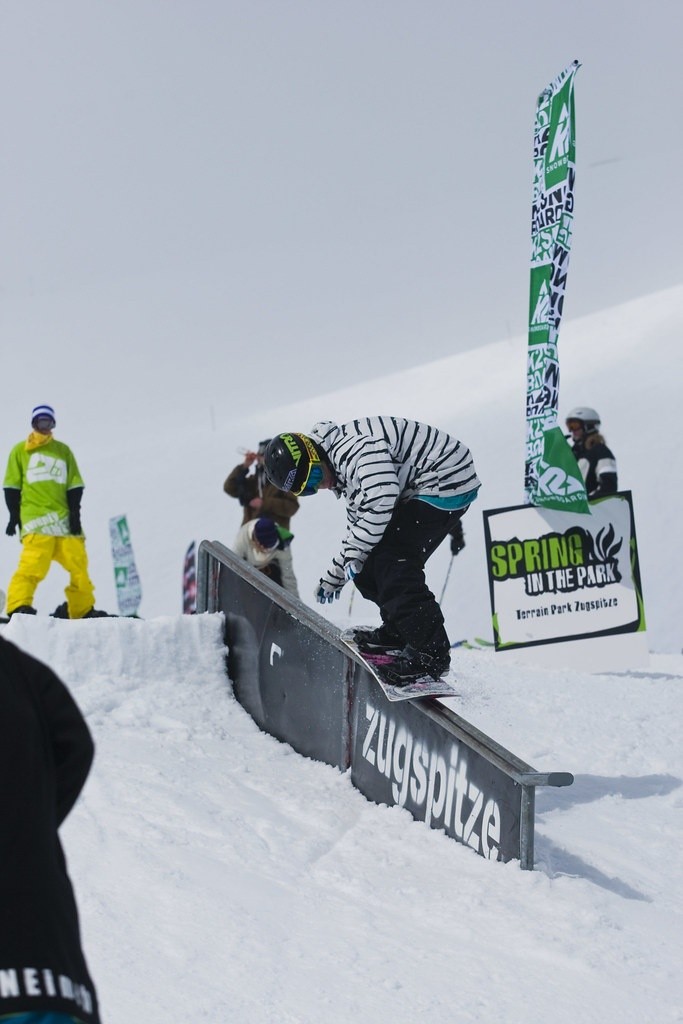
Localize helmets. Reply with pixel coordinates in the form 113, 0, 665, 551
566, 407, 601, 433
264, 432, 325, 497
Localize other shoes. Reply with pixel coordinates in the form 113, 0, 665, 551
83, 610, 107, 618
8, 605, 36, 617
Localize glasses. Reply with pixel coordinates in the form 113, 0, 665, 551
290, 432, 324, 497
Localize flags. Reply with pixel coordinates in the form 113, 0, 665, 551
524, 60, 594, 515
109, 515, 141, 617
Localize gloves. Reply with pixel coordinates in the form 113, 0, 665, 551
6, 519, 21, 536
314, 583, 341, 604
69, 514, 81, 535
343, 559, 362, 581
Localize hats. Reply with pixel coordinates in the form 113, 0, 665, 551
31, 405, 55, 428
255, 518, 278, 543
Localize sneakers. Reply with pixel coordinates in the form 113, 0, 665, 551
352, 622, 409, 650
377, 643, 451, 686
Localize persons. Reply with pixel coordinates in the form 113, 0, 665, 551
224, 438, 299, 598
565, 407, 617, 501
0, 635, 100, 1024
264, 415, 482, 687
3, 405, 107, 620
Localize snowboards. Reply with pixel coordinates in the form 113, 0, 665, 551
338, 624, 462, 703
